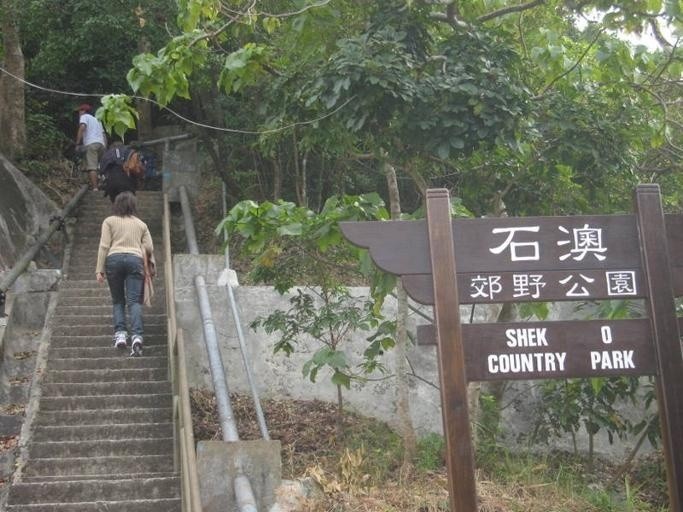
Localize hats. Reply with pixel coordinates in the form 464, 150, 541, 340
75, 104, 92, 112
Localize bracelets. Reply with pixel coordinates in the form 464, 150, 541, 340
76, 143, 82, 146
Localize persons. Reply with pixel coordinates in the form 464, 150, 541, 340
74, 104, 108, 193
94, 190, 154, 357
100, 141, 138, 202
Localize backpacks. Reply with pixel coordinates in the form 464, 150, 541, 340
124, 147, 147, 180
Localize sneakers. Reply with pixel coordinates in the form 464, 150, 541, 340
130, 335, 144, 357
114, 333, 127, 348
